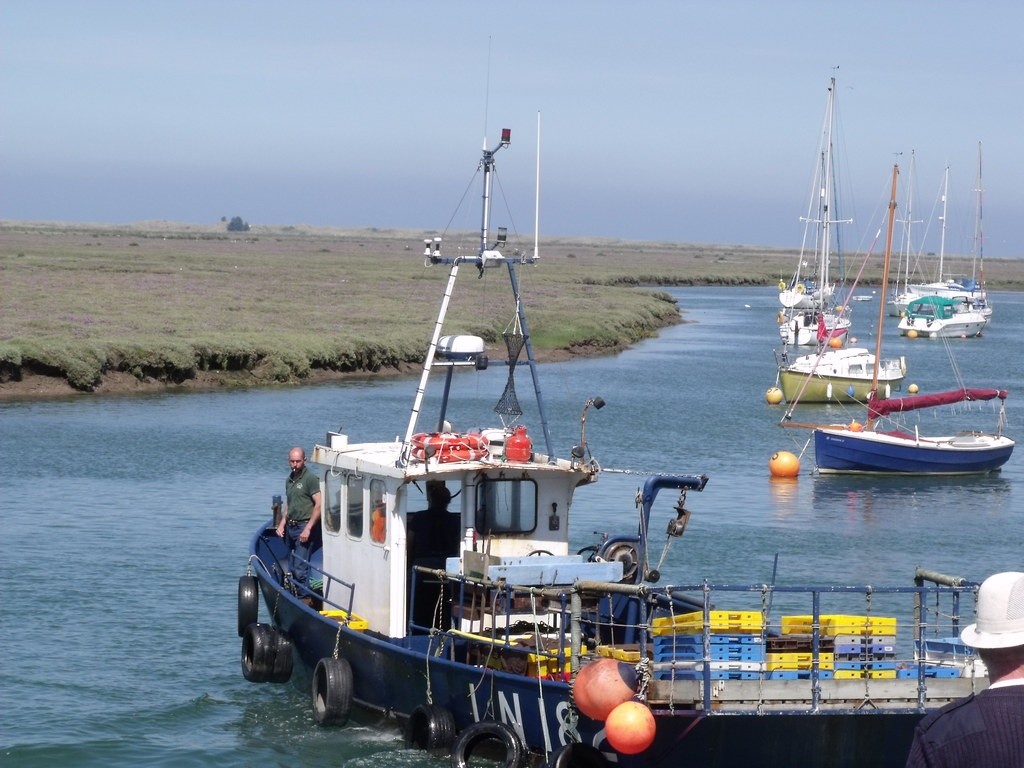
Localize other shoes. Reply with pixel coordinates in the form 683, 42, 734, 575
300, 597, 313, 606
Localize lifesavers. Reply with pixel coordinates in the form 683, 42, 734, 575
410, 448, 488, 462
411, 431, 489, 450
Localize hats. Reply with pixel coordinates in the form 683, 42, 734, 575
959, 572, 1024, 649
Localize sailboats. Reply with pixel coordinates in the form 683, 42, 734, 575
780, 143, 839, 308
891, 162, 990, 337
883, 140, 992, 313
775, 148, 1015, 475
766, 132, 907, 405
779, 77, 854, 348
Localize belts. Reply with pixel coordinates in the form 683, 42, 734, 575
286, 520, 310, 528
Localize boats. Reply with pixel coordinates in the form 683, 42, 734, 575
233, 108, 989, 768
852, 294, 873, 302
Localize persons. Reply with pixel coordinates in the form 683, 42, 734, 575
276, 446, 321, 611
905, 571, 1024, 768
372, 497, 385, 541
408, 484, 460, 598
